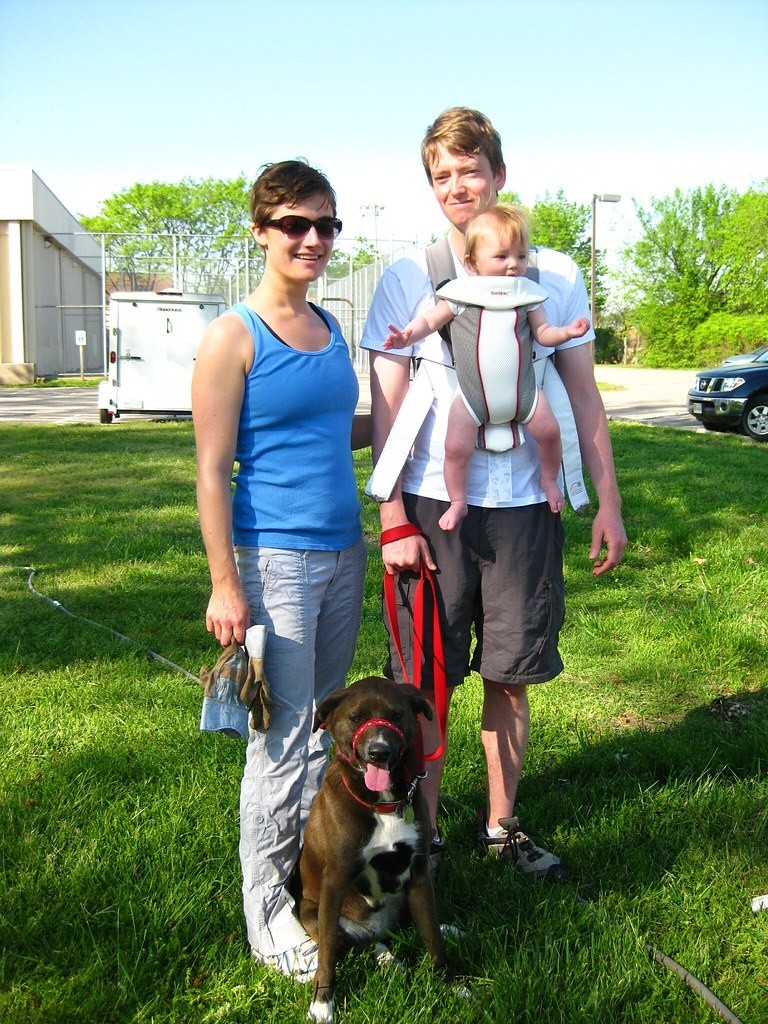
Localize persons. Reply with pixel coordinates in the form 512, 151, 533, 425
383, 205, 592, 532
193, 159, 374, 984
358, 107, 629, 880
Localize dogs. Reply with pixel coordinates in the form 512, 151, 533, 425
295, 673, 474, 1024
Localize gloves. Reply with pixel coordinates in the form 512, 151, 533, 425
200, 637, 252, 740
238, 625, 272, 731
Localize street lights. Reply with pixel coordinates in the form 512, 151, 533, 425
590, 194, 622, 371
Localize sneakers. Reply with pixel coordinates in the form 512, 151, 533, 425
249, 931, 319, 984
475, 817, 566, 880
426, 825, 445, 883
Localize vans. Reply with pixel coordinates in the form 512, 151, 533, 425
99, 287, 228, 425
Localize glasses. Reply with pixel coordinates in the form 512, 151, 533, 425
263, 215, 343, 240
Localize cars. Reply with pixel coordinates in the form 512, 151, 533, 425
721, 346, 768, 372
686, 351, 768, 442
